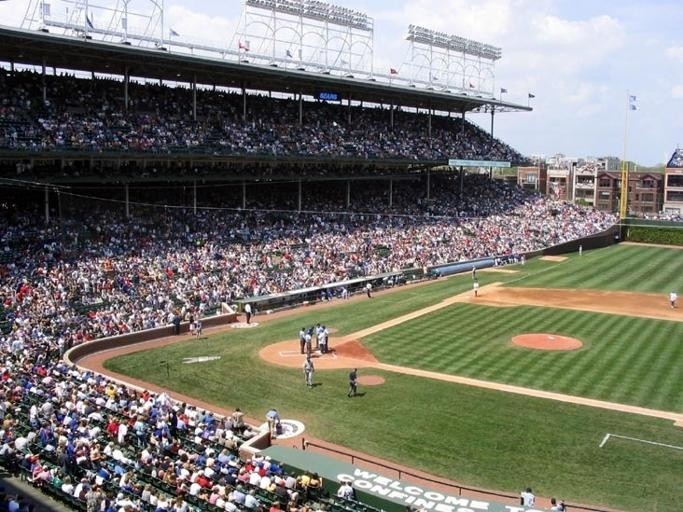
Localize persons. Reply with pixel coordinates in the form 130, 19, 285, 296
643, 211, 683, 222
0, 66, 620, 512
670, 291, 677, 309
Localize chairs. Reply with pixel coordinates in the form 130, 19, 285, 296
0, 67, 621, 512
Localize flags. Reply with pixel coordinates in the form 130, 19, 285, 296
240, 40, 250, 50
343, 58, 348, 64
287, 50, 293, 58
86, 15, 94, 29
170, 28, 180, 37
